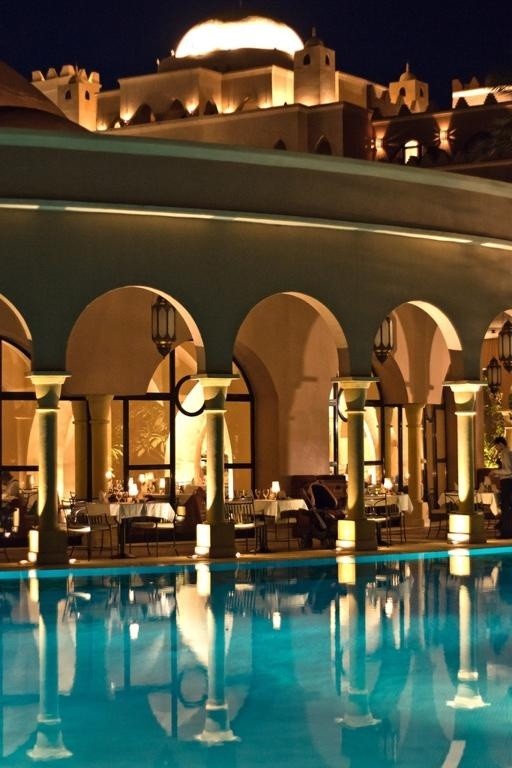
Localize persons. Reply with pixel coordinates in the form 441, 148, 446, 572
0, 471, 19, 528
490, 437, 512, 539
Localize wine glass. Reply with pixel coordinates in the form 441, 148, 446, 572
116, 491, 129, 504
243, 488, 269, 501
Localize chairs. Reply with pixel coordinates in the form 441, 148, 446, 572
224, 499, 266, 556
423, 488, 490, 538
61, 496, 179, 562
362, 492, 402, 548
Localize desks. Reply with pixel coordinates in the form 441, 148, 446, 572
440, 491, 494, 534
363, 492, 408, 543
232, 497, 306, 553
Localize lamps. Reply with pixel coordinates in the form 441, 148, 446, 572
373, 316, 394, 365
151, 294, 177, 359
485, 314, 511, 398
